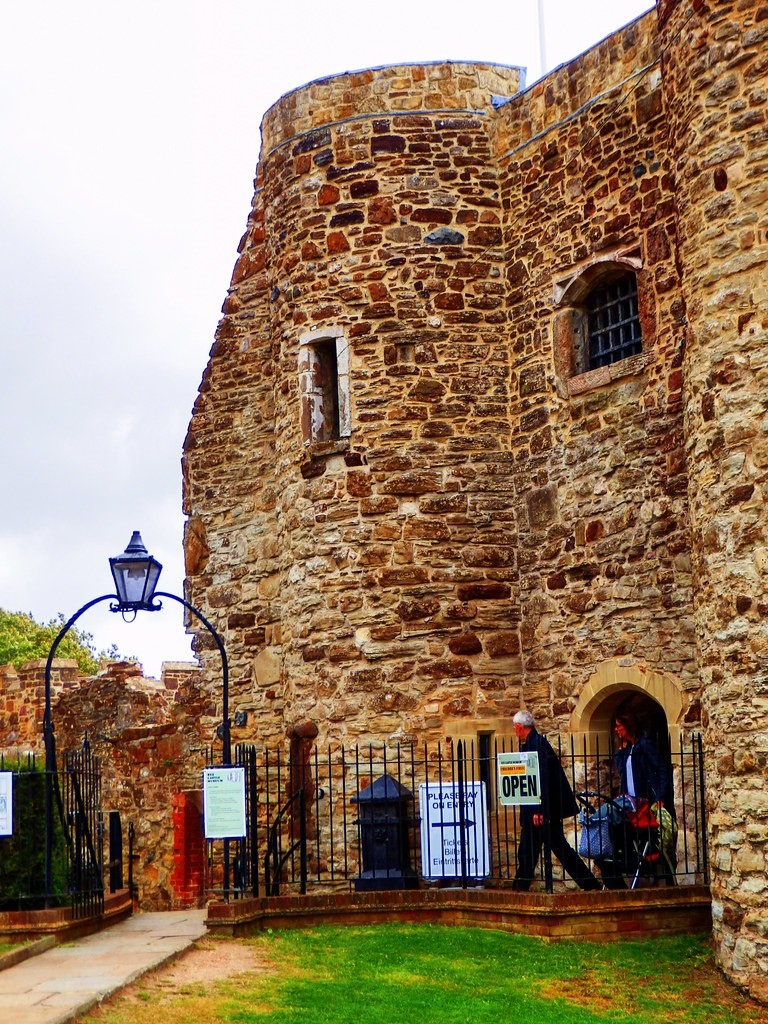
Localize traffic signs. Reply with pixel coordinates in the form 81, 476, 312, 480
419, 781, 490, 877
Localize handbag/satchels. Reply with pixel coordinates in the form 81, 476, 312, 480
651, 802, 678, 852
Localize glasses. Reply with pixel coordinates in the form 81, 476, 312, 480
615, 725, 624, 729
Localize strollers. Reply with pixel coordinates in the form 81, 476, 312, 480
574, 791, 677, 891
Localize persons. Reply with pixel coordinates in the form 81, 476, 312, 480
614, 712, 671, 889
509, 710, 608, 892
